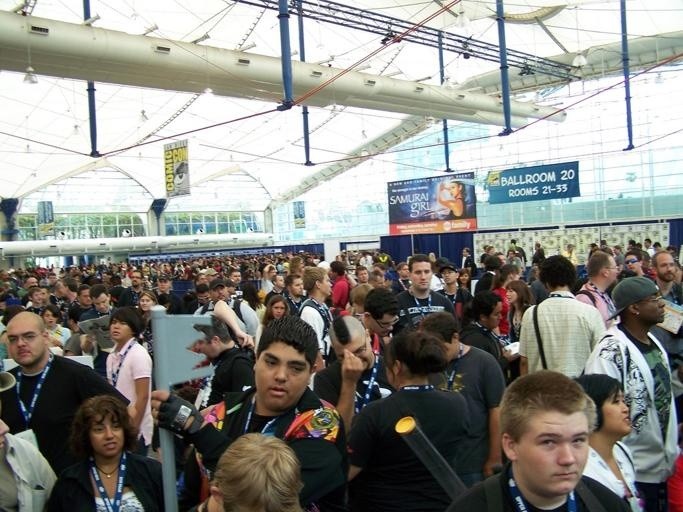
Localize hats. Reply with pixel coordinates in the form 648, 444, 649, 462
207, 278, 225, 290
437, 262, 458, 274
605, 275, 660, 323
203, 268, 219, 277
157, 273, 169, 281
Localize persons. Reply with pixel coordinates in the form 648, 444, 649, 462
438, 181, 466, 220
1, 241, 683, 512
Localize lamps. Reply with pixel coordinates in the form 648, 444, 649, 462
571, 7, 587, 69
457, 0, 469, 30
327, 62, 342, 114
20, 0, 41, 84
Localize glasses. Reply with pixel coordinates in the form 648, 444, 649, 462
332, 332, 367, 363
129, 275, 140, 280
648, 294, 663, 303
623, 257, 640, 265
373, 315, 400, 329
4, 331, 43, 345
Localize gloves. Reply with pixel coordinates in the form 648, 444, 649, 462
156, 392, 206, 438
